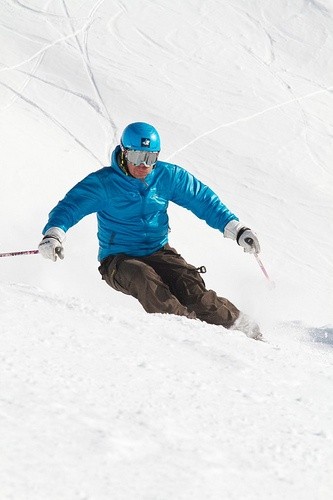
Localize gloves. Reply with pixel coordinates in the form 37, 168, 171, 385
223, 219, 260, 254
38, 227, 66, 262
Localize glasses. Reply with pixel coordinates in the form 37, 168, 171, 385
125, 151, 157, 167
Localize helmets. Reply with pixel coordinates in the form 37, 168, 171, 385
121, 122, 161, 152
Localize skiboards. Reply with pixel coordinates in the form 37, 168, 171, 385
254, 322, 333, 344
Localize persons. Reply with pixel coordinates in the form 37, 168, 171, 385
39, 121, 265, 344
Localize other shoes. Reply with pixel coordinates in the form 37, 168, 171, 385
229, 311, 265, 340
